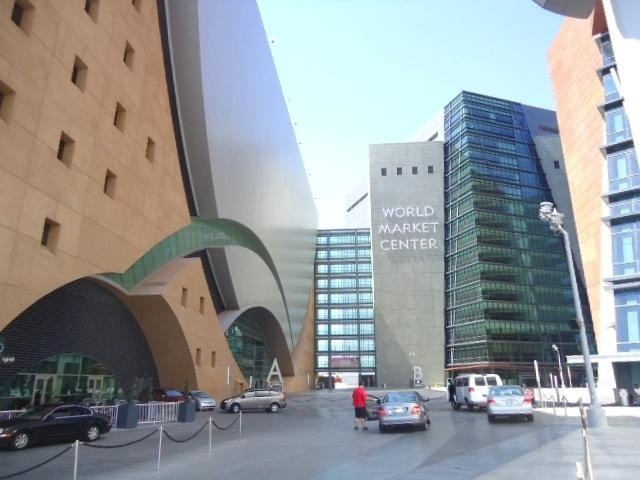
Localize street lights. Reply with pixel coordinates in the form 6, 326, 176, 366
538, 201, 599, 408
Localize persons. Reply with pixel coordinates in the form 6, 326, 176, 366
352, 384, 369, 430
632, 383, 640, 401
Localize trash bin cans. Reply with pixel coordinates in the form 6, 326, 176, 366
614, 387, 633, 407
115, 398, 138, 428
177, 395, 196, 423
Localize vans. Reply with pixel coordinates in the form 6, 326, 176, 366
446, 373, 504, 409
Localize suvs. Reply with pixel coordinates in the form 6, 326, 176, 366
220, 387, 287, 413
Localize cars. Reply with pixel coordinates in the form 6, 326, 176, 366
0, 403, 112, 450
138, 386, 216, 411
482, 385, 534, 422
365, 391, 431, 432
